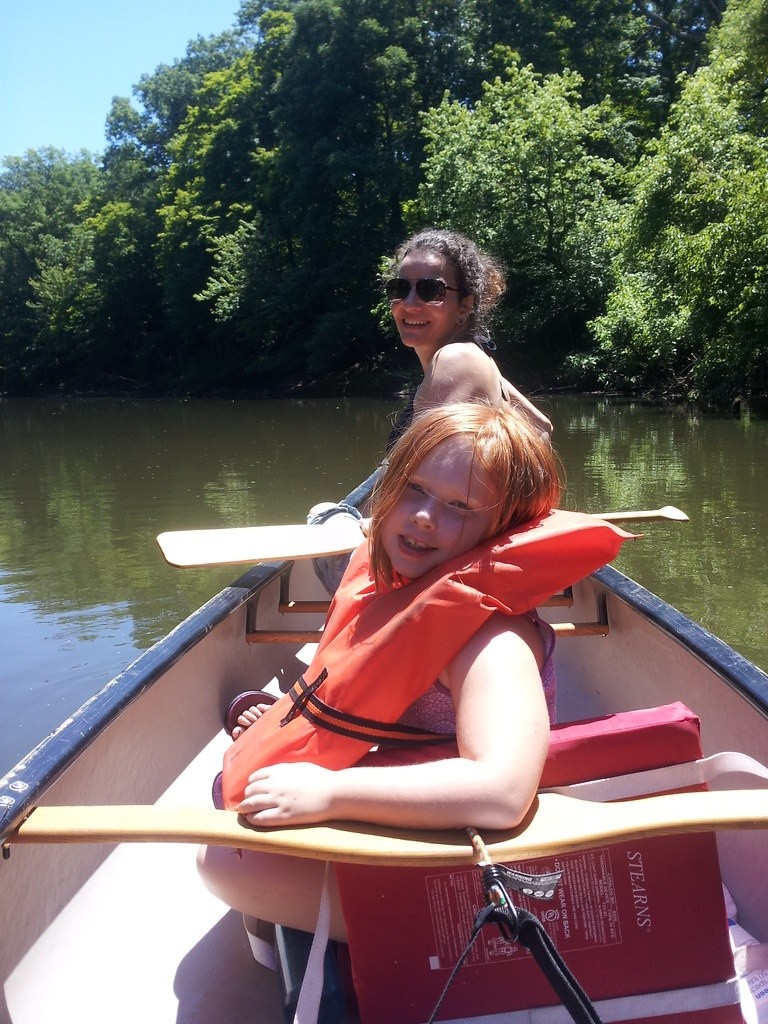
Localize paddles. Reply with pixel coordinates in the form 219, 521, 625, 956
157, 506, 689, 569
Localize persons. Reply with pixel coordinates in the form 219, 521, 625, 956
196, 231, 560, 943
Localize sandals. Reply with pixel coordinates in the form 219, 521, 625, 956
224, 691, 281, 742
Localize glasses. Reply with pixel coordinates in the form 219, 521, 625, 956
384, 279, 462, 304
